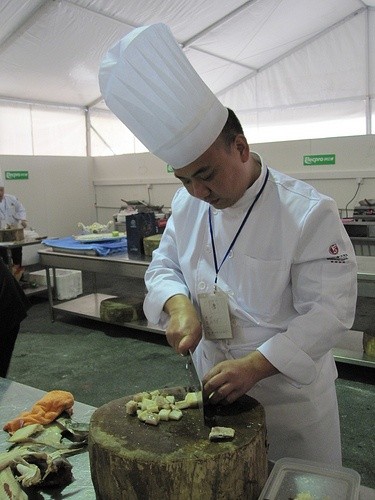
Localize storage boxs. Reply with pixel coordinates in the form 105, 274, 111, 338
143, 234, 164, 262
29, 269, 83, 301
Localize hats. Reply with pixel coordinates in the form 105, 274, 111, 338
98, 23, 229, 170
0, 168, 4, 187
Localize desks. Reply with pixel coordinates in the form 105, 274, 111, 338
37, 248, 375, 369
0, 236, 59, 304
0, 377, 375, 500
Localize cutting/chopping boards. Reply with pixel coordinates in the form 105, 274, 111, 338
99, 296, 145, 323
87, 385, 267, 500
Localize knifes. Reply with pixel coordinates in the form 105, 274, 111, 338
180, 350, 206, 430
